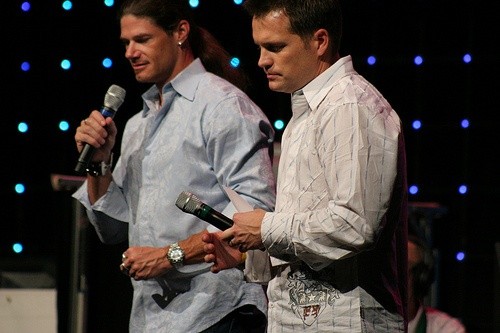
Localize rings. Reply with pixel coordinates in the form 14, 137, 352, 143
121, 263, 129, 271
230, 239, 241, 247
122, 253, 127, 259
84, 118, 88, 125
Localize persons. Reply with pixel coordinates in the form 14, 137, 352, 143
75, 0, 276, 333
202, 0, 404, 333
407, 215, 467, 333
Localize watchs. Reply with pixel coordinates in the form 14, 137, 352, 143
167, 241, 185, 269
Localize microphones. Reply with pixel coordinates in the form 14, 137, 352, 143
73, 84, 126, 177
175, 191, 234, 231
